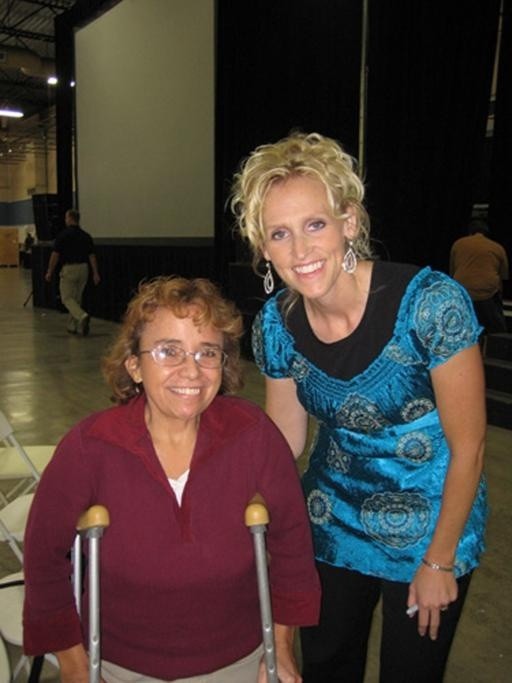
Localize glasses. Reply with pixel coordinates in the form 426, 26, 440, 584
133, 341, 231, 369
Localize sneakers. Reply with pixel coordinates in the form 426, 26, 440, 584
67, 313, 90, 335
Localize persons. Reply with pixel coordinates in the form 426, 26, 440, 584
21, 274, 323, 683
24, 232, 35, 251
45, 209, 102, 337
448, 222, 508, 334
242, 126, 491, 683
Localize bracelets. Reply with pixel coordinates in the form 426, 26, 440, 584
421, 559, 453, 573
